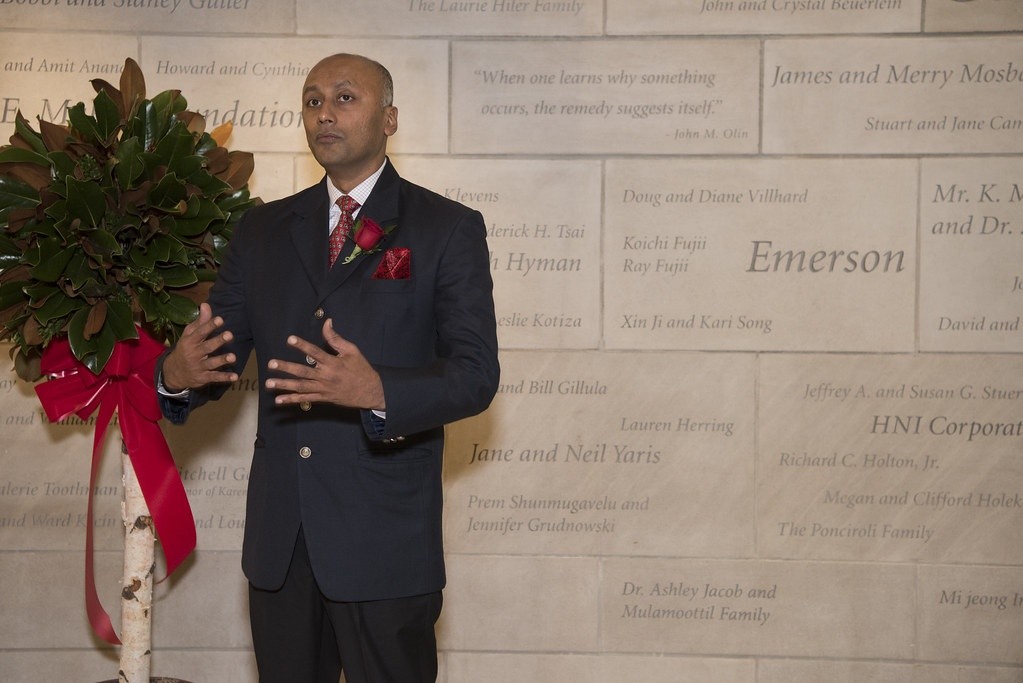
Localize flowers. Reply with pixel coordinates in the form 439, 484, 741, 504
341, 213, 398, 265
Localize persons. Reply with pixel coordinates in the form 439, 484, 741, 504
155, 54, 503, 683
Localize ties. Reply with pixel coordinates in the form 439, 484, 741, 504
329, 196, 361, 272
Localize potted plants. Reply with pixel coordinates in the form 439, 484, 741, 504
0, 54, 270, 683
